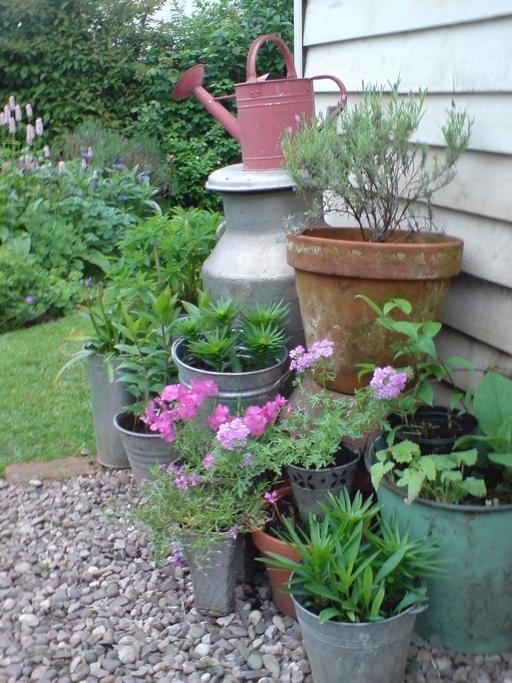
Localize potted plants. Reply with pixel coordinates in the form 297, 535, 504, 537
105, 282, 511, 655
277, 80, 475, 398
254, 485, 453, 683
65, 253, 188, 469
